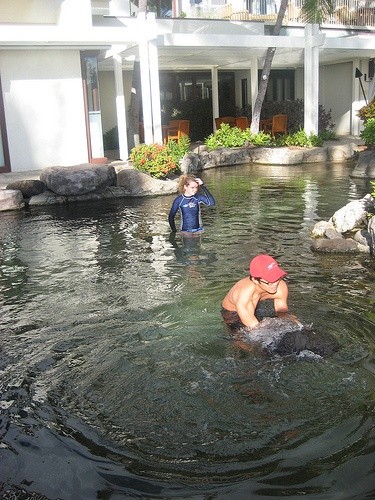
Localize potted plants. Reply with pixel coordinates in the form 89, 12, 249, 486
168, 108, 190, 139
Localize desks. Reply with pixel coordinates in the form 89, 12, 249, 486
139, 125, 177, 145
248, 120, 272, 131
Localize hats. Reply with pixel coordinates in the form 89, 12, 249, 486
250, 255, 287, 283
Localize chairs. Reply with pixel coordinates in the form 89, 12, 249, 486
215, 115, 287, 137
163, 130, 180, 144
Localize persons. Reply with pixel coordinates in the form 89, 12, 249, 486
168, 174, 215, 233
220, 255, 300, 332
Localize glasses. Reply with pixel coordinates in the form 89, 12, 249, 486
259, 280, 272, 286
